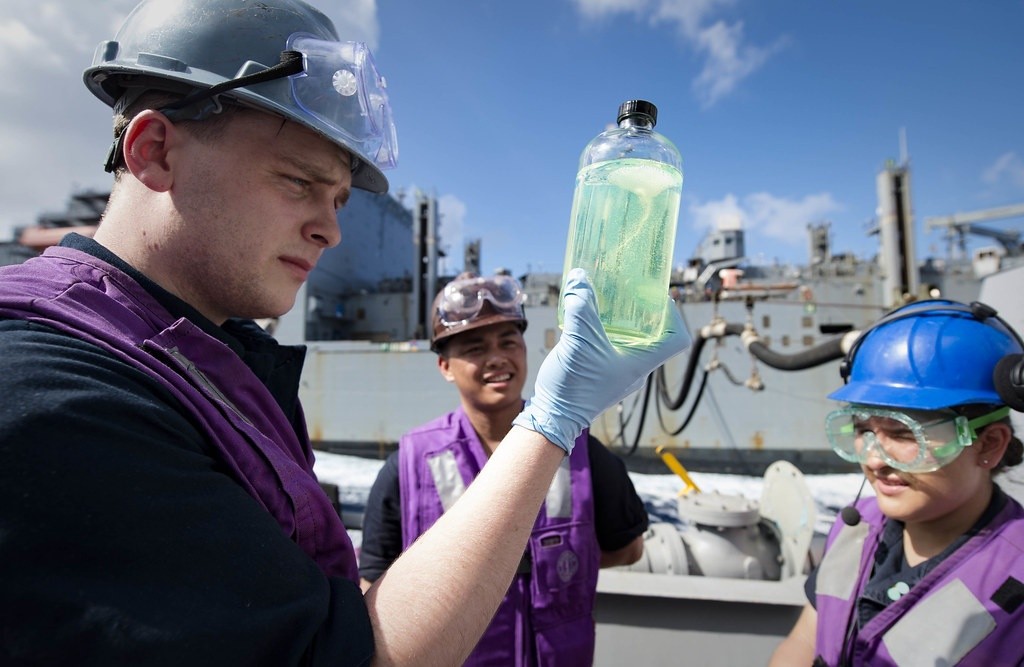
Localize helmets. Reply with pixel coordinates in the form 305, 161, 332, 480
82, 0, 390, 196
429, 272, 530, 353
827, 299, 1024, 413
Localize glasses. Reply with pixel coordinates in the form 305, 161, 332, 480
825, 405, 973, 472
434, 275, 528, 326
287, 32, 399, 169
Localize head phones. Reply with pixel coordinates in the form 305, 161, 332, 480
839, 302, 1024, 413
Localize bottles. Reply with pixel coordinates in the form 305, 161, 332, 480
557, 100, 684, 345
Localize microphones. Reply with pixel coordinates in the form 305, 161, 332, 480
841, 476, 872, 527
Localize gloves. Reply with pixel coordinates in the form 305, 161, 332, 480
510, 269, 692, 455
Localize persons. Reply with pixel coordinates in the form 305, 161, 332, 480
0, 0, 692, 667
358, 272, 650, 667
766, 299, 1024, 667
